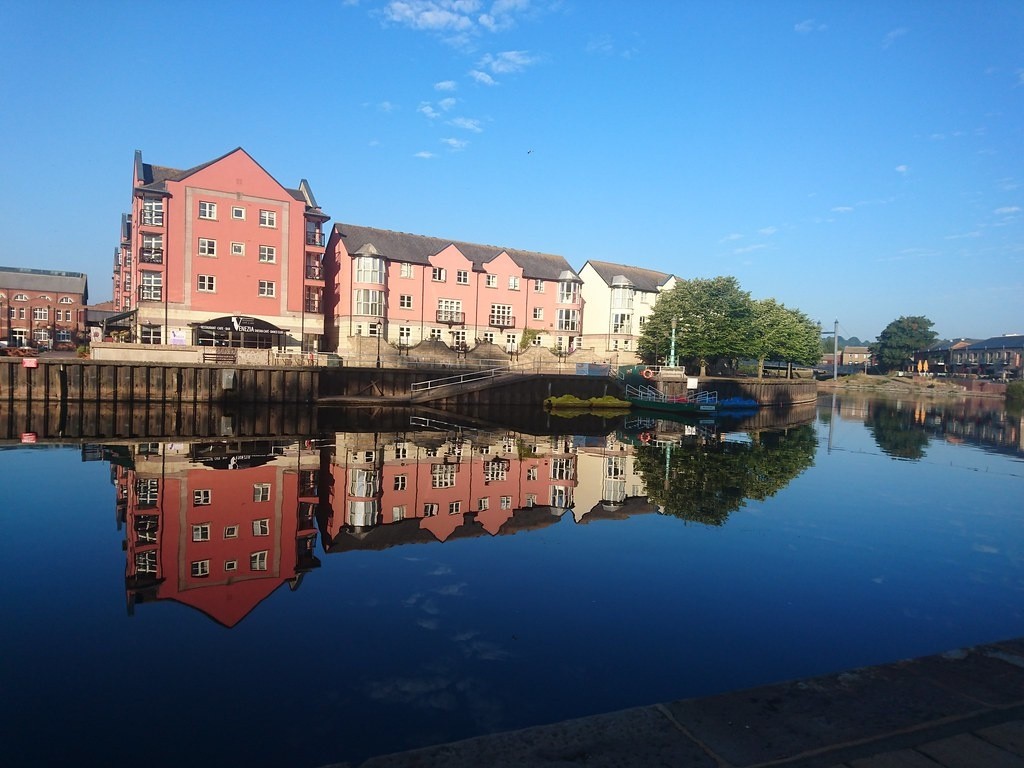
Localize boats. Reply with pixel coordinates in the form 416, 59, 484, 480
587, 395, 632, 407
590, 408, 631, 419
543, 408, 589, 419
543, 394, 592, 408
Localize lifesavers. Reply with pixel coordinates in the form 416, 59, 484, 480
644, 368, 652, 378
640, 432, 651, 441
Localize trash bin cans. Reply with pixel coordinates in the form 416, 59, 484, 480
327, 355, 339, 368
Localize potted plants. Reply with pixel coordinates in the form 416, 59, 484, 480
77, 345, 90, 358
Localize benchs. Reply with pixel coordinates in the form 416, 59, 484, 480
657, 365, 685, 378
203, 353, 237, 364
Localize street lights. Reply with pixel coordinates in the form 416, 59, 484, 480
668, 314, 684, 366
376, 319, 382, 367
